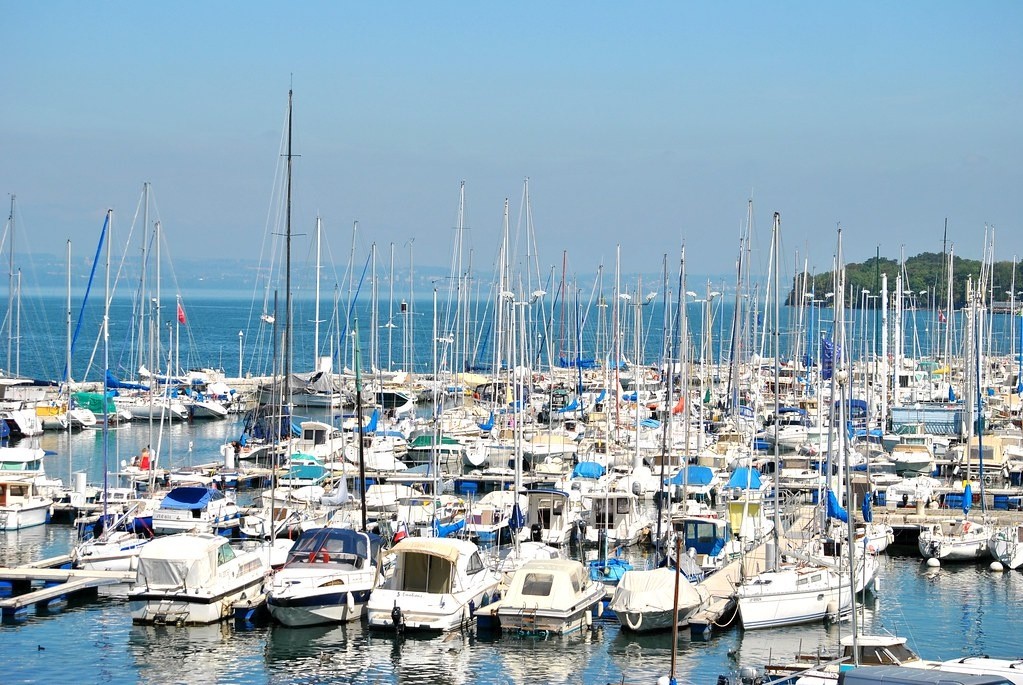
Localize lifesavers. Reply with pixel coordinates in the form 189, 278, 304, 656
309, 548, 329, 563
472, 393, 480, 399
653, 374, 659, 380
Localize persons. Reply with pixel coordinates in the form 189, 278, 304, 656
131, 445, 155, 471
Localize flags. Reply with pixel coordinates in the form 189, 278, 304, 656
703, 389, 710, 402
673, 397, 684, 414
938, 311, 947, 323
179, 305, 185, 325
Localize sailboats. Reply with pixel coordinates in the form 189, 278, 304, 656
0, 71, 1023, 685
